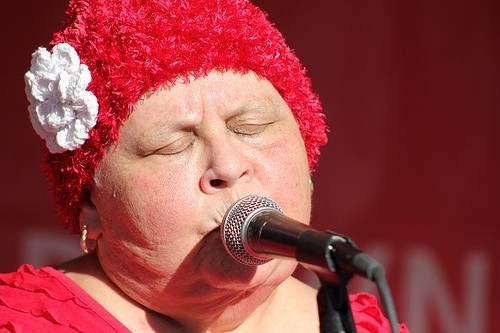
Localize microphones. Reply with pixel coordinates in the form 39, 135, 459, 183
220, 194, 385, 282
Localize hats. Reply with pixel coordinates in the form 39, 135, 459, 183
25, 0, 330, 234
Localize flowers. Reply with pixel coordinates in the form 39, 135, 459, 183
23, 43, 99, 154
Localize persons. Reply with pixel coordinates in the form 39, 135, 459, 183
0, 0, 411, 333
